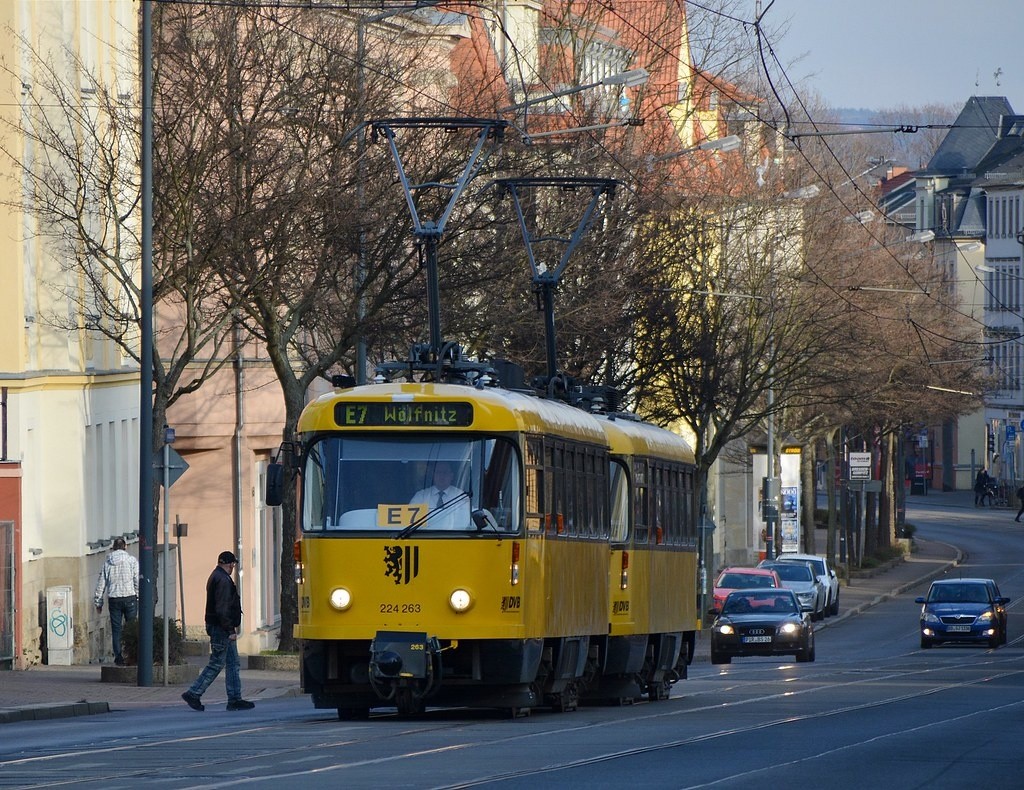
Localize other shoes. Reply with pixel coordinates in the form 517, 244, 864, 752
182, 690, 205, 712
226, 700, 255, 711
1015, 519, 1021, 522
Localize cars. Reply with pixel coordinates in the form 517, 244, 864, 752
707, 588, 816, 664
915, 577, 1012, 649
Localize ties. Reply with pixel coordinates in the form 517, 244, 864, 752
436, 491, 446, 510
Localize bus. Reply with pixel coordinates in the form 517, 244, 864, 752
264, 118, 705, 721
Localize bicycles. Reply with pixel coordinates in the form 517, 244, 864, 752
981, 480, 1009, 508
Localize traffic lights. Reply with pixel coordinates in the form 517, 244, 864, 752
988, 434, 995, 452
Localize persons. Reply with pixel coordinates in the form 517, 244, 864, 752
180, 551, 256, 713
1014, 484, 1024, 522
974, 468, 991, 506
405, 460, 470, 510
774, 598, 787, 611
94, 538, 140, 665
737, 598, 752, 613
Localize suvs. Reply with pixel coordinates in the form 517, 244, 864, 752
710, 564, 784, 622
755, 559, 825, 622
776, 552, 839, 620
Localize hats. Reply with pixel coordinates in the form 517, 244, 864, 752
218, 551, 239, 564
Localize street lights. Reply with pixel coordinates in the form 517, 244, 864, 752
605, 134, 743, 385
765, 209, 874, 561
